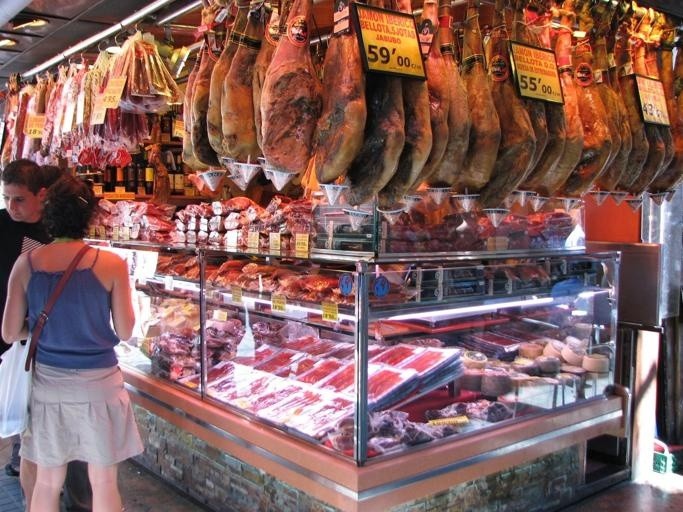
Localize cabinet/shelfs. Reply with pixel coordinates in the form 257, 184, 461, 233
80, 237, 633, 511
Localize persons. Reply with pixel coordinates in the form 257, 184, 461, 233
1, 176, 144, 512
41, 165, 59, 189
0, 158, 92, 512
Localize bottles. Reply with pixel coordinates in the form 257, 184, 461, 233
74, 148, 197, 197
